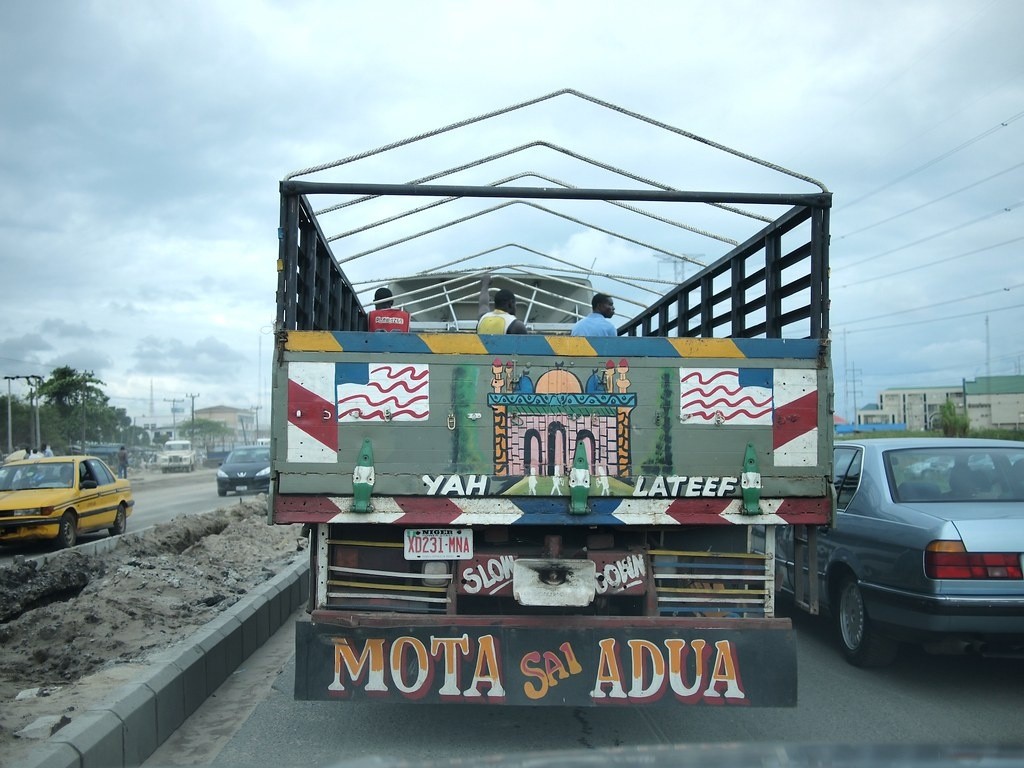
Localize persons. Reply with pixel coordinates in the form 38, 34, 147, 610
362, 288, 410, 333
24, 443, 54, 460
476, 289, 527, 335
58, 466, 72, 487
571, 291, 618, 337
118, 445, 129, 479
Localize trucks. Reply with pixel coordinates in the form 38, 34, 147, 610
262, 175, 838, 708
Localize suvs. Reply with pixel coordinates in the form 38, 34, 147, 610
160, 440, 196, 473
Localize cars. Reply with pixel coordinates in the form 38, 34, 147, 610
750, 439, 1024, 668
0, 456, 135, 548
215, 445, 270, 496
